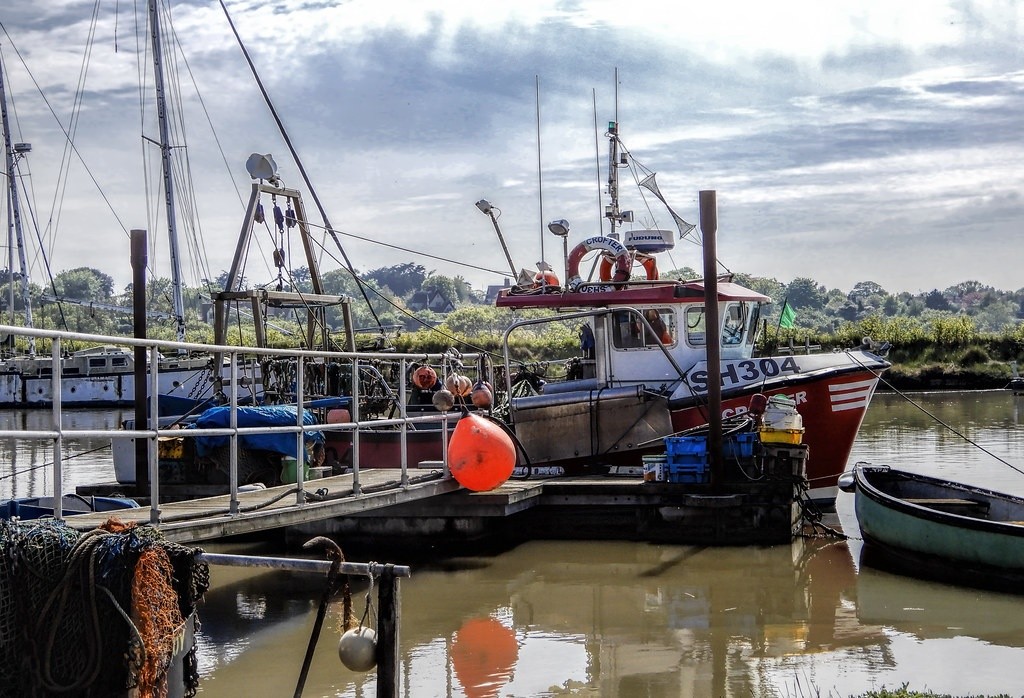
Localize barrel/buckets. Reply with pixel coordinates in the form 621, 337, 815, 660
642, 455, 668, 482
282, 455, 310, 483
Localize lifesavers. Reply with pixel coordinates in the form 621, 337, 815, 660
565, 234, 633, 293
599, 248, 660, 289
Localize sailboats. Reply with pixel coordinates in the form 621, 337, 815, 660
0, 0, 296, 409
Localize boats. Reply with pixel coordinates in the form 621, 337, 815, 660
110, 68, 893, 515
846, 562, 1023, 644
833, 458, 1023, 580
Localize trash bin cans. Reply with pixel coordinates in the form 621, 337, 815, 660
663, 433, 753, 484
